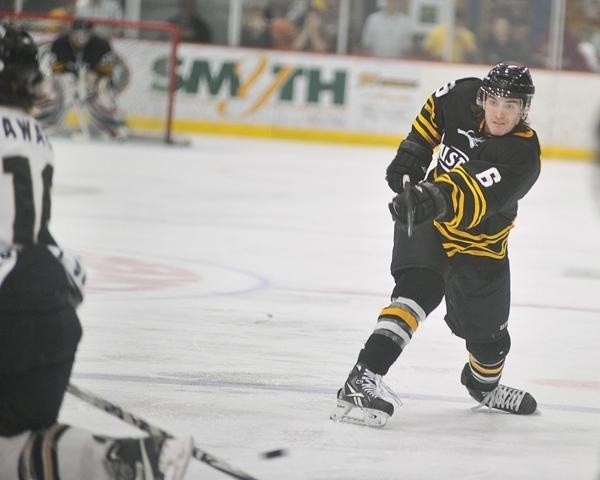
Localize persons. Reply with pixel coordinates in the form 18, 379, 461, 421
336, 61, 543, 416
0, 20, 195, 480
37, 0, 599, 75
29, 15, 132, 141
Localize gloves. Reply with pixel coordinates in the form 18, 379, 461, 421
389, 180, 447, 232
384, 140, 434, 195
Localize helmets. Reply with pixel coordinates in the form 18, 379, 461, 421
479, 60, 535, 111
0, 24, 43, 105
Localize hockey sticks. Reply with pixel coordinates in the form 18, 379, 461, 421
403, 173, 417, 237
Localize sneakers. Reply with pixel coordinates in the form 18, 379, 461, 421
467, 382, 537, 416
336, 363, 395, 419
91, 430, 181, 480
